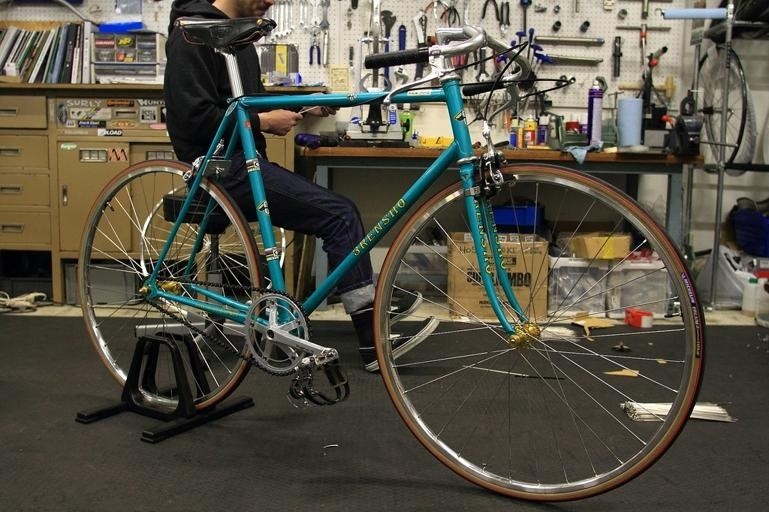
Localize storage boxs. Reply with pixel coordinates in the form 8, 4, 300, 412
446, 204, 668, 324
64, 261, 144, 305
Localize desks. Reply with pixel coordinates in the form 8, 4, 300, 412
300, 143, 705, 317
0, 82, 331, 306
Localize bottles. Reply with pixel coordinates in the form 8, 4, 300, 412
566, 121, 589, 135
509, 116, 550, 147
587, 80, 604, 146
741, 277, 758, 319
399, 104, 414, 142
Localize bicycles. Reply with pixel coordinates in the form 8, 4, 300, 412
75, 16, 707, 502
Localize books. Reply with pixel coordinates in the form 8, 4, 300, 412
0, 21, 100, 85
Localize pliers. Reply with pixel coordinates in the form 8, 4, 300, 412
310, 35, 320, 64
482, 0, 510, 37
424, 0, 462, 28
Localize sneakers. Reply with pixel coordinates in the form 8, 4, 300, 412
358, 290, 440, 372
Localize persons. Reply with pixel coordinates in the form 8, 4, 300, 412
164, 0, 441, 373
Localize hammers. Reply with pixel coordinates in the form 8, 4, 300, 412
618, 75, 673, 98
